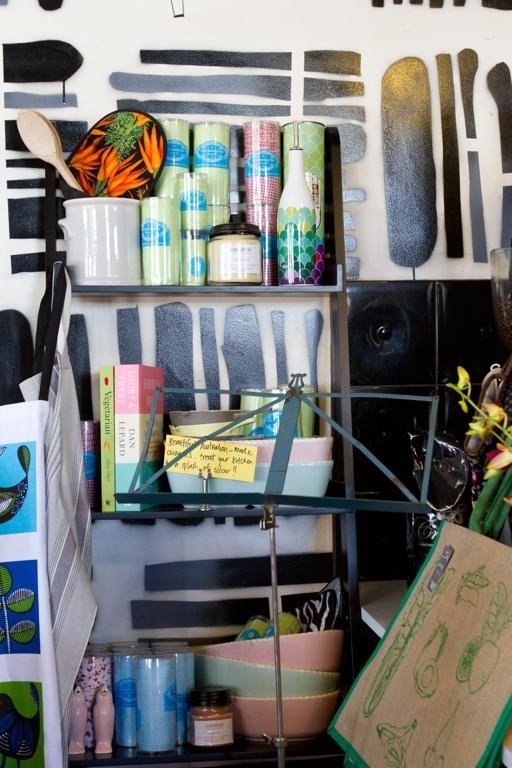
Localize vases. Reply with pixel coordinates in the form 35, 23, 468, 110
276, 149, 316, 286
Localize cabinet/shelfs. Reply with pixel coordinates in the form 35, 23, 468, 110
69, 264, 362, 766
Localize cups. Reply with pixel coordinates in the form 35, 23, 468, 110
54, 198, 141, 289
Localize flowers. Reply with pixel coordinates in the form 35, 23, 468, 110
447, 363, 510, 537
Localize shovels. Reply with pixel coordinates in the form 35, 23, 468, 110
16, 109, 86, 192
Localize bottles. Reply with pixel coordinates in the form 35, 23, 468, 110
237, 610, 298, 639
138, 114, 232, 287
242, 117, 326, 287
241, 387, 317, 438
77, 419, 100, 507
74, 638, 196, 756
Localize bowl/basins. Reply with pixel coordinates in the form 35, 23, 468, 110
166, 407, 272, 438
201, 630, 350, 748
